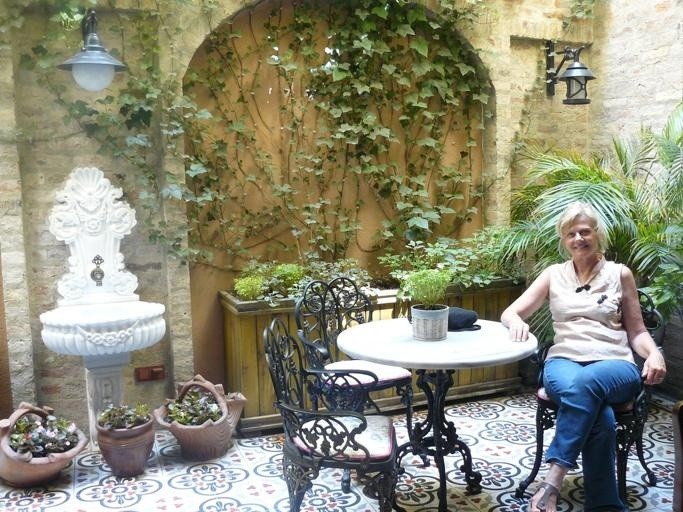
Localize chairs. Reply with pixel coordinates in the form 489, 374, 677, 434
669, 398, 681, 512
513, 286, 668, 508
285, 273, 431, 494
253, 313, 402, 511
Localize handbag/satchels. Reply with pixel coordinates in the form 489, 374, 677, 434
447, 305, 482, 332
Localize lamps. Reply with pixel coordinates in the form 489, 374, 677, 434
541, 37, 598, 107
49, 5, 132, 95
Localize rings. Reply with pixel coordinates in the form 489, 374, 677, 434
657, 377, 664, 380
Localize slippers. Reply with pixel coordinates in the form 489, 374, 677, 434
526, 481, 561, 512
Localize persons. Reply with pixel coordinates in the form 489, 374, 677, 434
500, 200, 667, 512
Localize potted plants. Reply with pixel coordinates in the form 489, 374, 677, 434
396, 267, 451, 344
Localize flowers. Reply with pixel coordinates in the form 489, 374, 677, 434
6, 412, 79, 457
93, 396, 151, 434
162, 387, 222, 426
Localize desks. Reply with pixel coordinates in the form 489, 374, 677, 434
330, 305, 536, 510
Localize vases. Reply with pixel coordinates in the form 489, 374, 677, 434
151, 372, 248, 461
93, 410, 157, 477
0, 399, 90, 489
